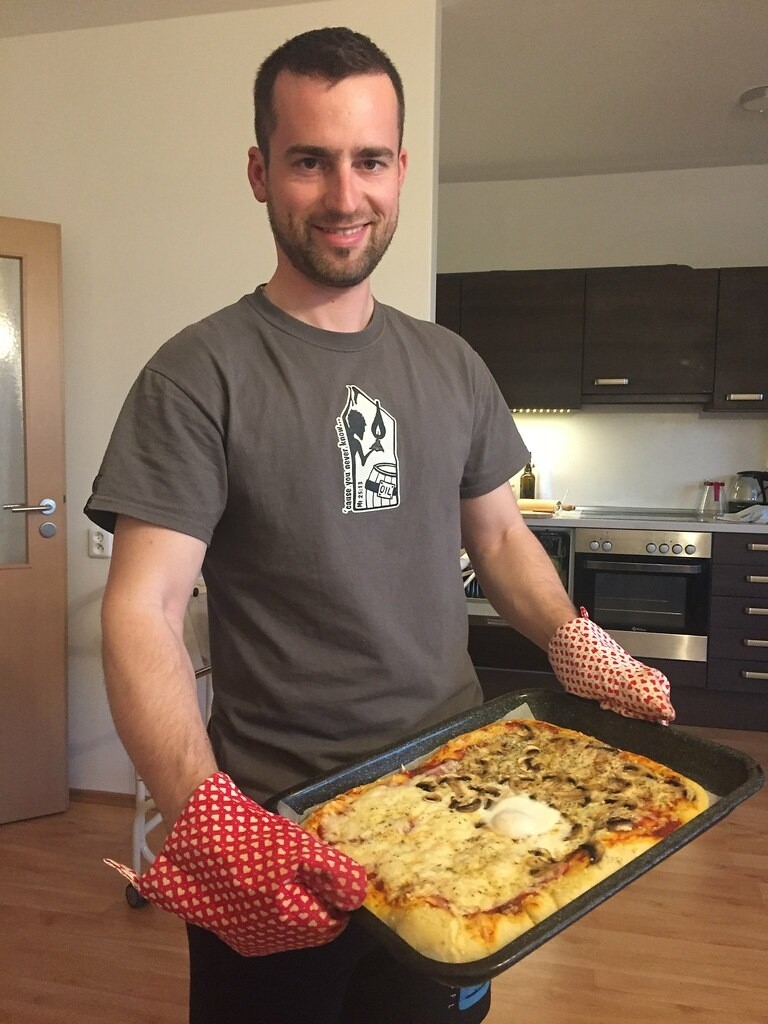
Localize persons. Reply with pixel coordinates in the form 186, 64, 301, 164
83, 26, 678, 1024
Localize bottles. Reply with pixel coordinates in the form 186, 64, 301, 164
520, 452, 535, 499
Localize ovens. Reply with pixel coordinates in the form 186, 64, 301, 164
574, 527, 712, 661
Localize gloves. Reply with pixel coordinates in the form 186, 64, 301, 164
547, 606, 676, 727
105, 772, 368, 957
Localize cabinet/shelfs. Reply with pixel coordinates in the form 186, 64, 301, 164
713, 267, 768, 410
581, 268, 719, 394
461, 272, 586, 410
707, 531, 768, 694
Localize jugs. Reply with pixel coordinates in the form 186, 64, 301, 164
697, 479, 728, 523
729, 471, 768, 513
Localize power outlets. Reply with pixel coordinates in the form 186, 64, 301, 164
88, 527, 113, 559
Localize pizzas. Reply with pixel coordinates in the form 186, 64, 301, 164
298, 719, 708, 962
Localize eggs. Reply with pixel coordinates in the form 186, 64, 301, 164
475, 795, 560, 838
